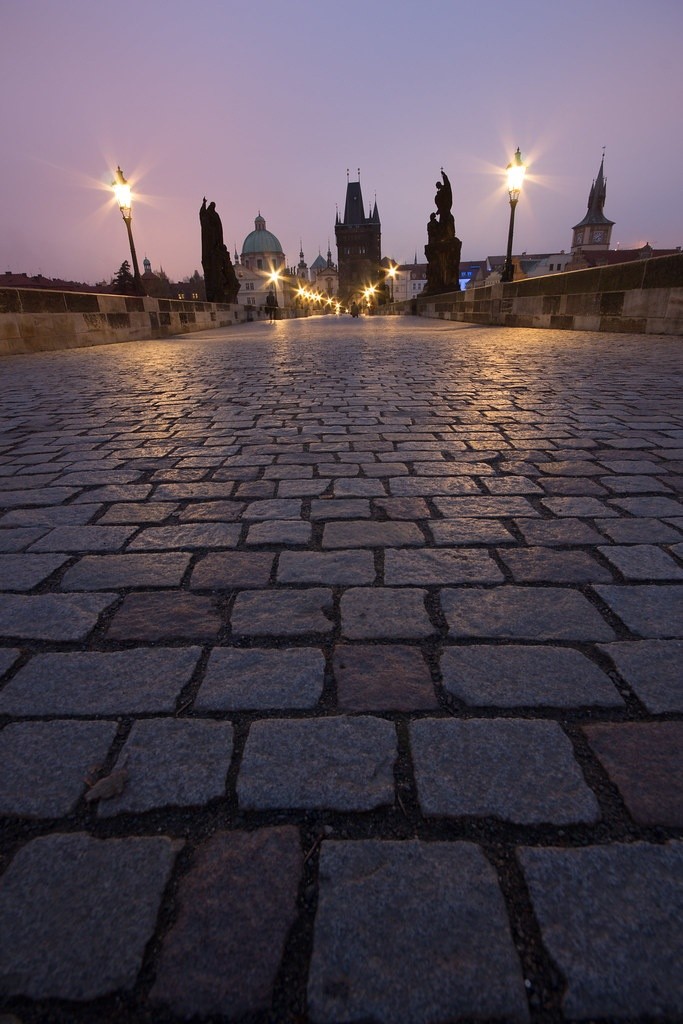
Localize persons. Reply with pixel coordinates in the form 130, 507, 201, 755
351, 302, 360, 318
427, 170, 455, 245
410, 295, 417, 315
266, 290, 279, 320
199, 196, 242, 303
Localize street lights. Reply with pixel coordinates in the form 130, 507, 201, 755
500, 147, 527, 283
272, 271, 279, 307
389, 267, 396, 302
112, 165, 147, 296
